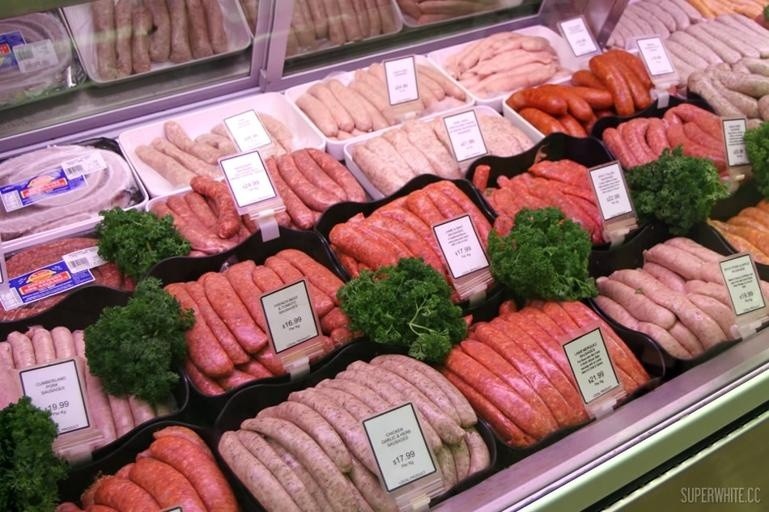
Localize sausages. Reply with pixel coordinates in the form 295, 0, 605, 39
2, 0, 769, 511
